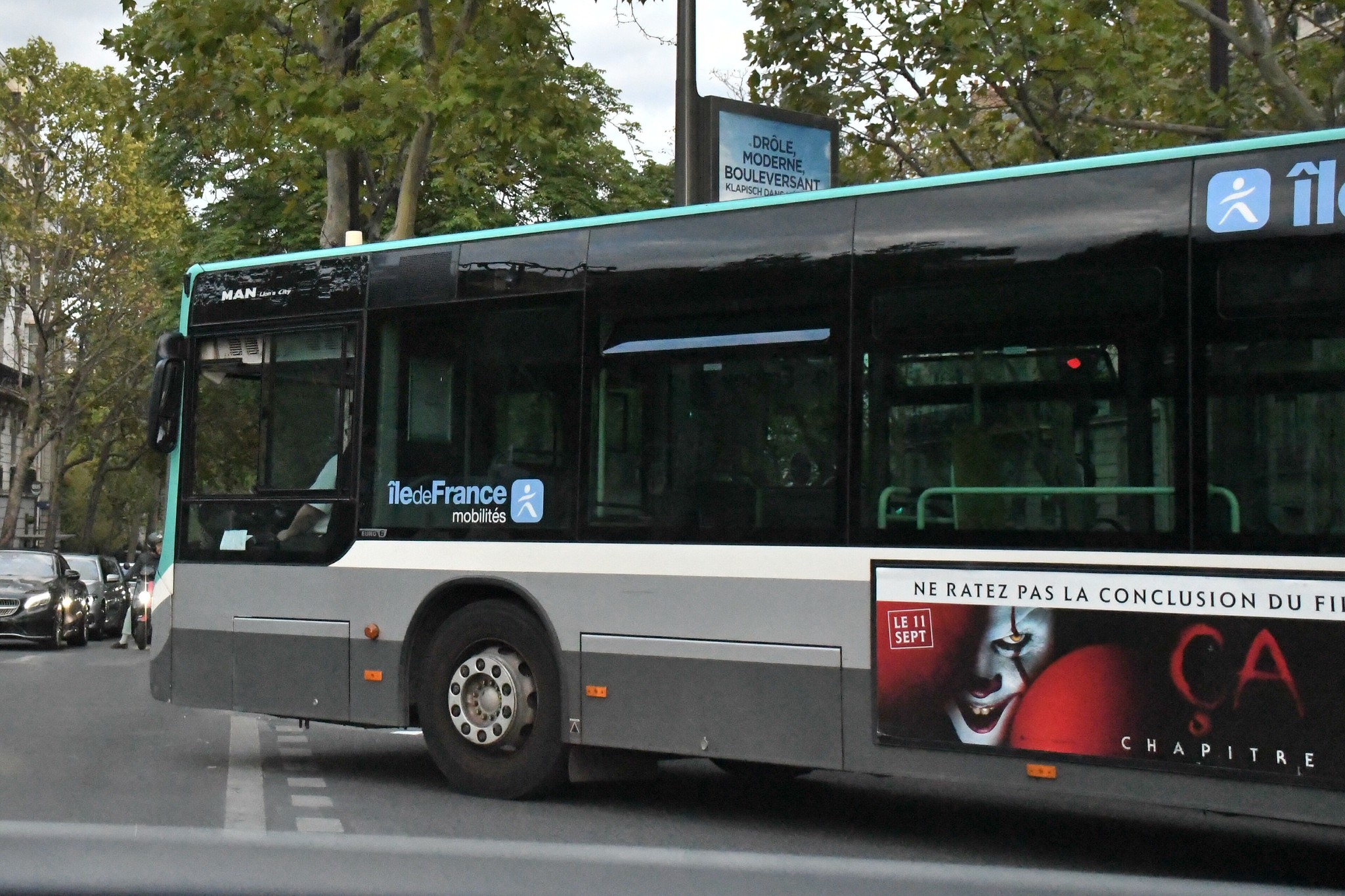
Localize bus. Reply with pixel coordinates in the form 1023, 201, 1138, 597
145, 124, 1344, 859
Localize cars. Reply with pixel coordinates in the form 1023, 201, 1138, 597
0, 547, 136, 649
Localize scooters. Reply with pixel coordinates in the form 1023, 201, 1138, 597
131, 564, 153, 648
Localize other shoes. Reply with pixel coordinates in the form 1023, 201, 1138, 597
110, 641, 129, 649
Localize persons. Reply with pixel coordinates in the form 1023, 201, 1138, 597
112, 533, 162, 650
277, 455, 337, 550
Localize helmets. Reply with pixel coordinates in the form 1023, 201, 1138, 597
146, 532, 164, 551
